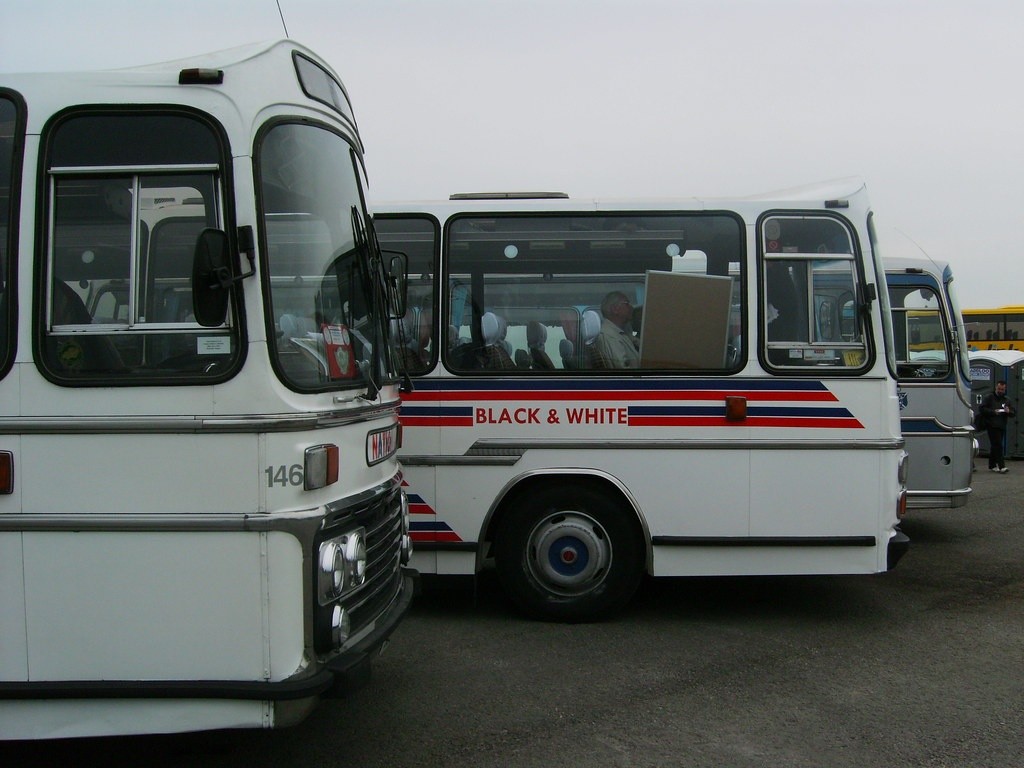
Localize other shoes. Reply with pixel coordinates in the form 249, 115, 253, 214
999, 467, 1010, 474
992, 467, 1001, 473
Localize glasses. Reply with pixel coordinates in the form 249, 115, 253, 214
618, 301, 632, 305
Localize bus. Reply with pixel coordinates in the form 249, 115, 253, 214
83, 256, 980, 513
130, 192, 911, 619
0, 37, 415, 742
905, 304, 1024, 367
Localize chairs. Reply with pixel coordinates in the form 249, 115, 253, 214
986, 329, 992, 340
1008, 329, 1012, 339
281, 315, 310, 371
580, 311, 613, 370
514, 348, 531, 371
481, 312, 517, 372
974, 332, 980, 340
966, 330, 973, 340
526, 320, 555, 371
559, 339, 577, 369
1014, 331, 1018, 339
993, 331, 999, 340
297, 317, 325, 371
504, 340, 513, 357
388, 315, 422, 373
460, 337, 477, 371
447, 325, 459, 370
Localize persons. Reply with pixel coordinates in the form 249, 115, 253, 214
594, 290, 641, 368
978, 380, 1015, 472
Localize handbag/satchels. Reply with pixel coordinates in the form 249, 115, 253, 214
974, 413, 987, 433
